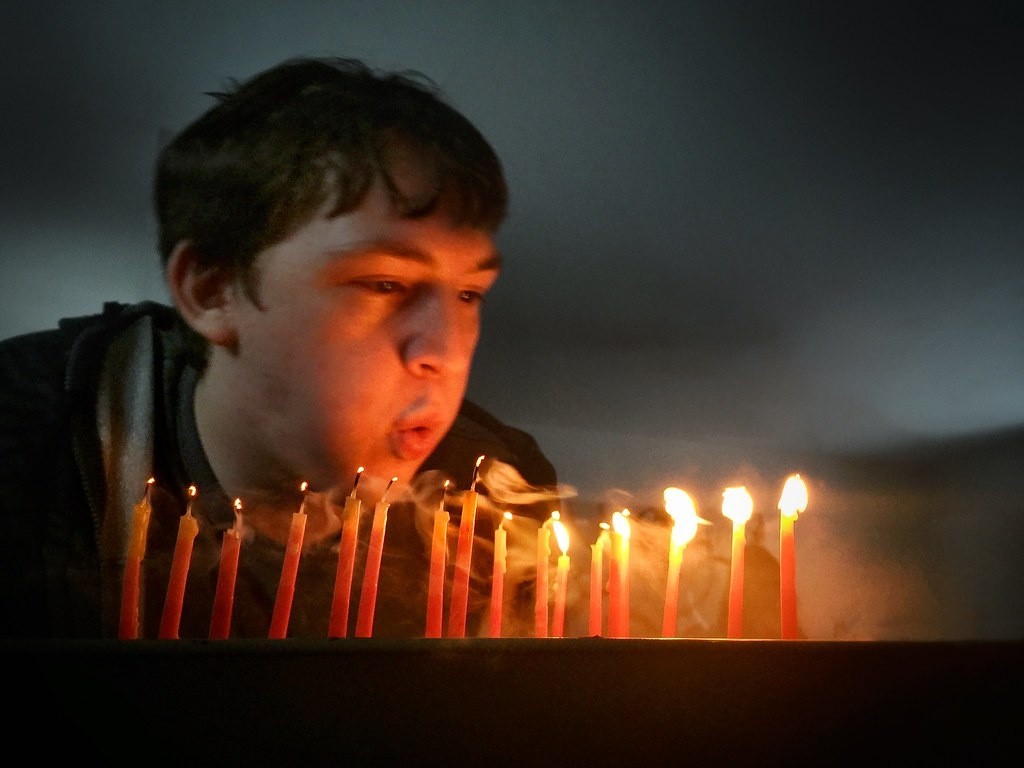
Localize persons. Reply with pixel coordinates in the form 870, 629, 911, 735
1, 56, 561, 642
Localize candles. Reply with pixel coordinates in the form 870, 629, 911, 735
117, 457, 809, 640
